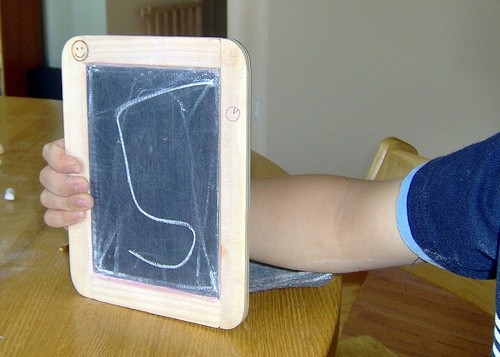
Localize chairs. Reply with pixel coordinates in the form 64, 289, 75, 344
323, 135, 497, 357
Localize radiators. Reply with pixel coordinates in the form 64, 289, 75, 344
140, 3, 203, 37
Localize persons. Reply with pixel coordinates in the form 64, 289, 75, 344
40, 132, 500, 357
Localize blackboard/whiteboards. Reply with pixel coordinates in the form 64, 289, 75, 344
56, 36, 256, 329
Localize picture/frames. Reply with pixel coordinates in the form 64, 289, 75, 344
62, 34, 252, 328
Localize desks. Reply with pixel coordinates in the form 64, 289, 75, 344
0, 95, 341, 357
